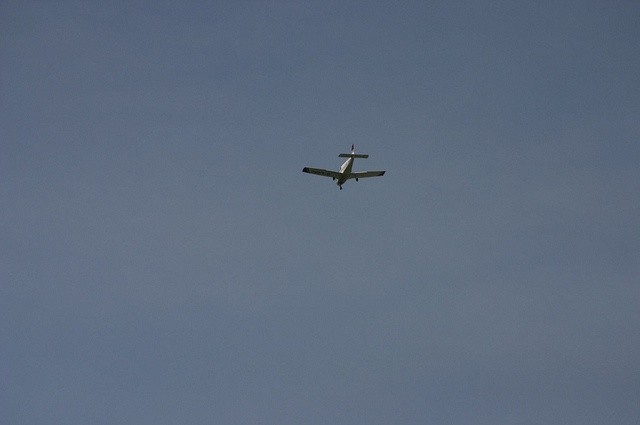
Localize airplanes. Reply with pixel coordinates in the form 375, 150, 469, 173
302, 143, 385, 189
